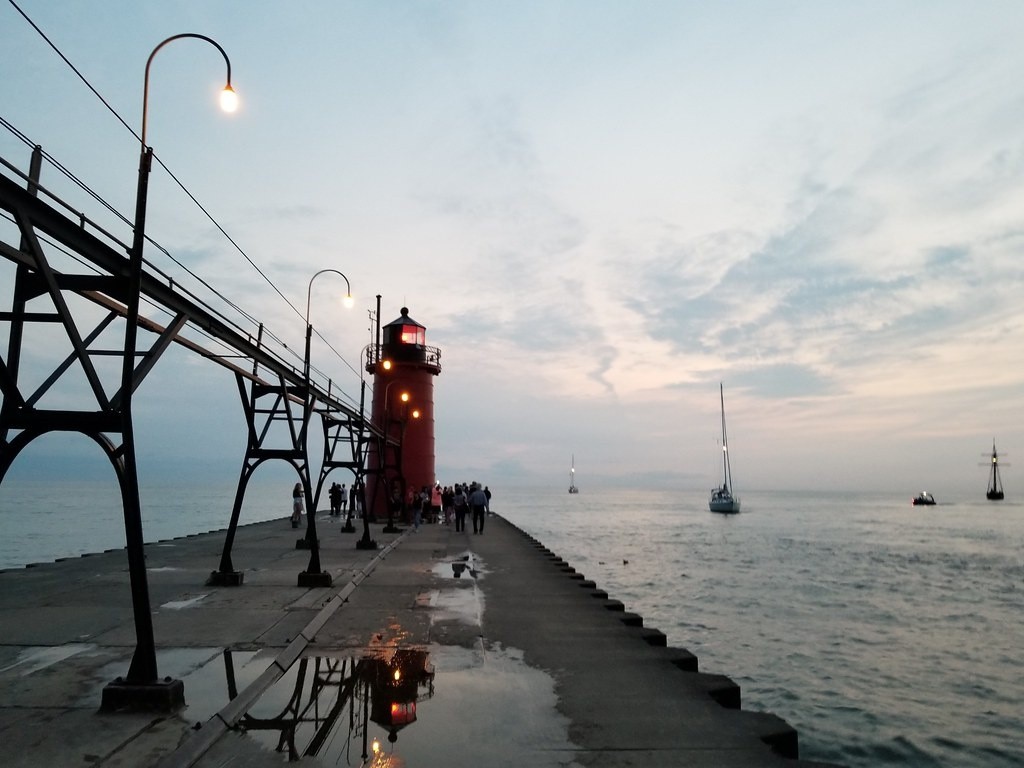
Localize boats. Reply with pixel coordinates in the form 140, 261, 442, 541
912, 491, 936, 505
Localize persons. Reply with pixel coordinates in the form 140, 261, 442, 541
291, 483, 306, 523
465, 484, 488, 535
391, 481, 491, 523
411, 490, 423, 533
329, 482, 365, 518
453, 487, 467, 534
452, 555, 469, 579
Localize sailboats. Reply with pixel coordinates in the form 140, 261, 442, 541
567, 453, 578, 493
985, 438, 1005, 502
708, 381, 740, 513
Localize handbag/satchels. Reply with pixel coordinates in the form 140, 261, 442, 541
463, 494, 471, 513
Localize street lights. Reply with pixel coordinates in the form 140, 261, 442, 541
144, 33, 241, 149
305, 268, 352, 379
399, 401, 419, 424
360, 342, 390, 403
383, 378, 409, 419
